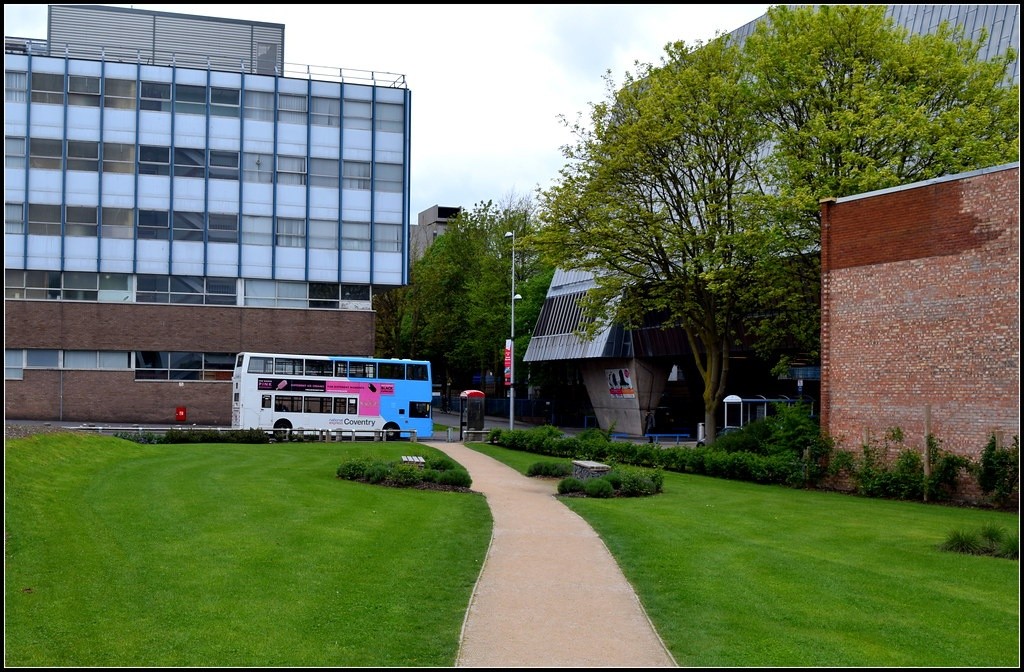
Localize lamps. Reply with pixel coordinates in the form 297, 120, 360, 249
513, 293, 522, 301
504, 231, 514, 239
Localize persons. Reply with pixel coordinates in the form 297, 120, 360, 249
441, 394, 448, 414
645, 410, 658, 443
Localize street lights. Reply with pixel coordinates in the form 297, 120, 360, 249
506, 229, 522, 431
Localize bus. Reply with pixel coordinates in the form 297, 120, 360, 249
231, 351, 434, 441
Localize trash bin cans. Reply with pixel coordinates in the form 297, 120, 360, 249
696, 422, 706, 440
